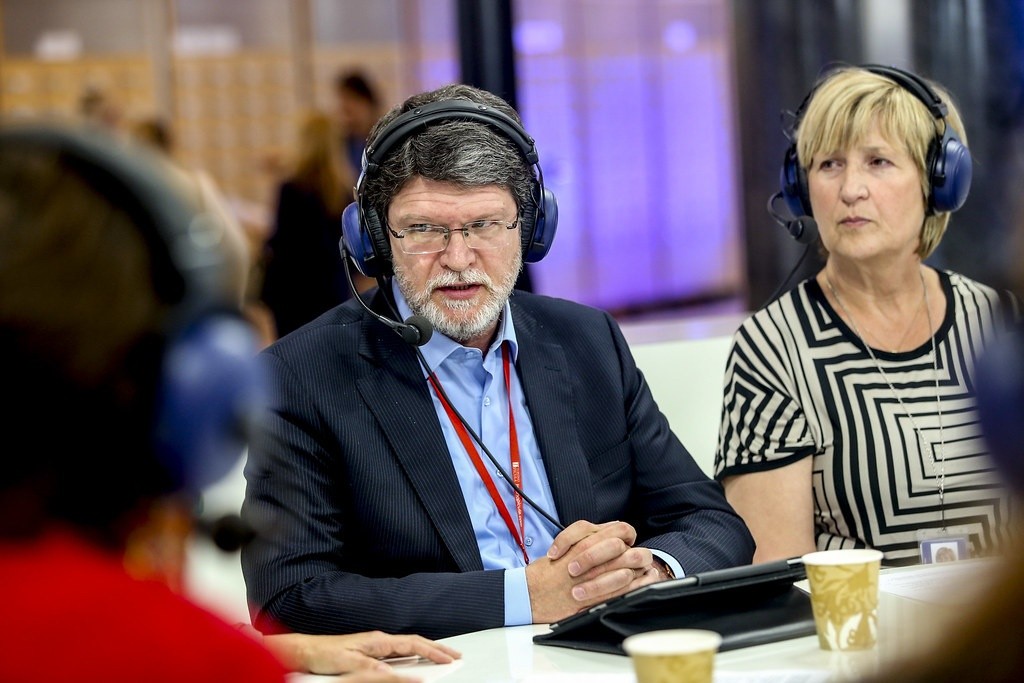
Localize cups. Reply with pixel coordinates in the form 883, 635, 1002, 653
801, 549, 883, 653
620, 628, 720, 682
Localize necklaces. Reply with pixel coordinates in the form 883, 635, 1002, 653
822, 265, 946, 501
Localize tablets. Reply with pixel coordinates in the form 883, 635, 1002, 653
549, 558, 806, 631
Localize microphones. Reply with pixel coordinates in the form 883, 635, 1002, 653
174, 499, 254, 553
340, 248, 432, 347
766, 192, 819, 244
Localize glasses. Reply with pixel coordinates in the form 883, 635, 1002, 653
381, 207, 521, 255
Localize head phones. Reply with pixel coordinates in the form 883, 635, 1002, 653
340, 100, 558, 278
3, 117, 268, 488
779, 64, 972, 220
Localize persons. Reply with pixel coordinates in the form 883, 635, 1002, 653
0, 59, 462, 682
709, 60, 1024, 585
234, 83, 760, 649
